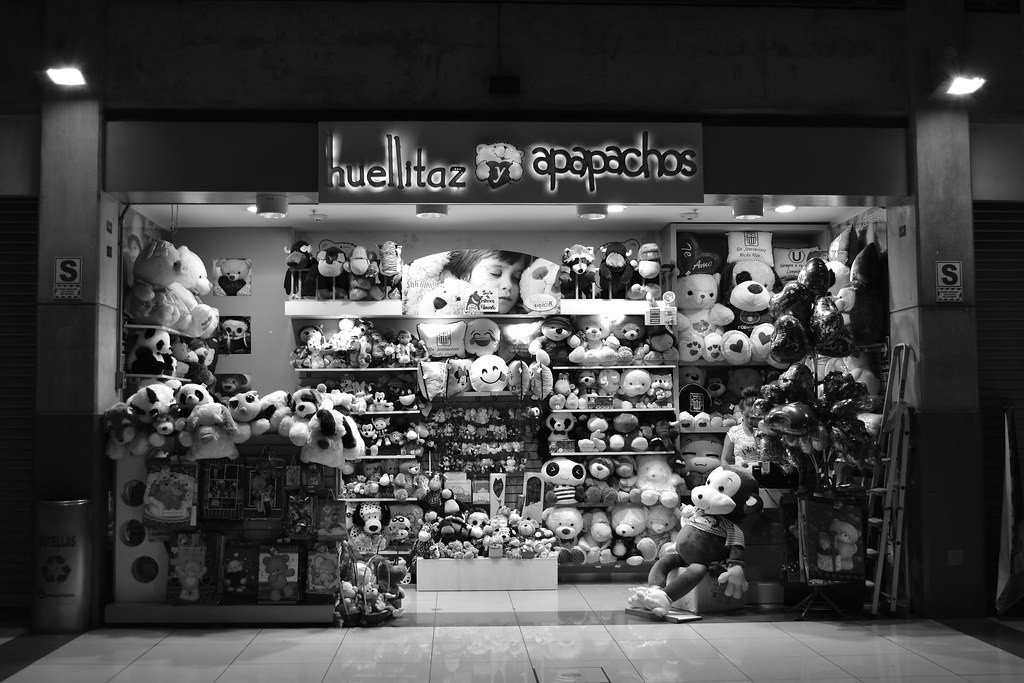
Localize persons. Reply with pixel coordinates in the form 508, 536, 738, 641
128, 231, 141, 262
445, 250, 533, 313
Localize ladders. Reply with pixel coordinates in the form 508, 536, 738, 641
859, 342, 910, 613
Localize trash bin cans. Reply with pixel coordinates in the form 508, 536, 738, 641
33, 495, 96, 634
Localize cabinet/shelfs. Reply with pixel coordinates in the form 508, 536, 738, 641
123, 223, 830, 625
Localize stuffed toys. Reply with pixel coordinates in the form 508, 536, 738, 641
102, 222, 881, 619
212, 374, 250, 398
216, 315, 251, 355
213, 258, 252, 296
